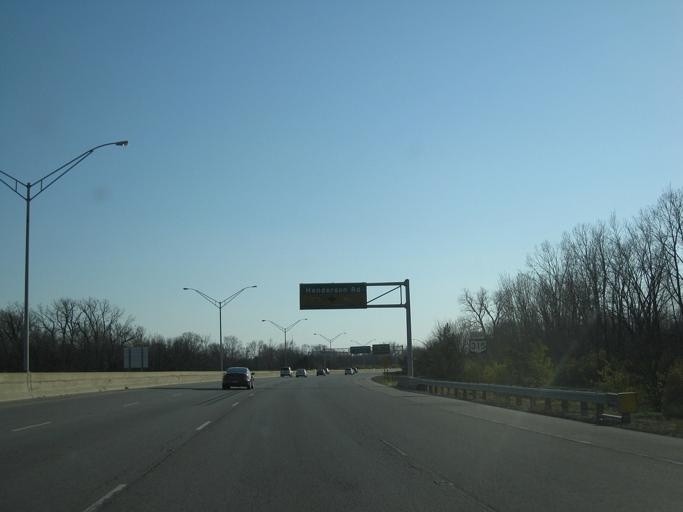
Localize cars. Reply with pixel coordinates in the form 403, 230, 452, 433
345, 368, 358, 375
280, 366, 330, 377
223, 366, 255, 389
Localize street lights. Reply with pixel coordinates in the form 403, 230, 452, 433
312, 331, 347, 369
182, 285, 258, 371
350, 338, 376, 368
261, 318, 308, 365
0, 140, 129, 373
